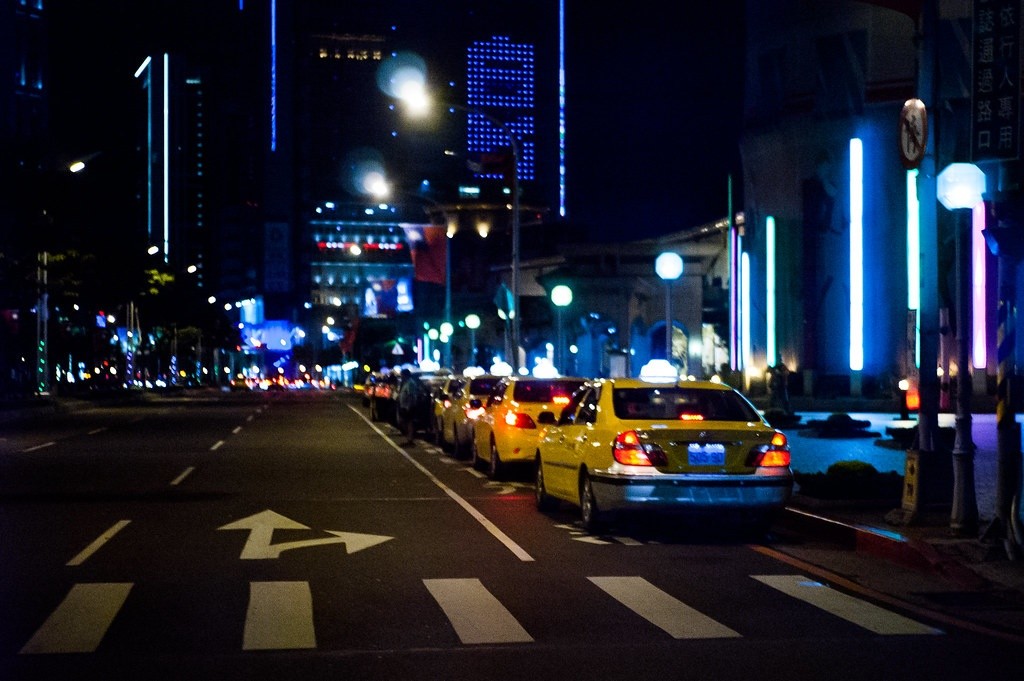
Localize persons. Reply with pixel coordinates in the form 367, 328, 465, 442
768, 353, 794, 415
719, 364, 740, 389
393, 369, 417, 448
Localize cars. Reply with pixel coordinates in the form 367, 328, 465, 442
363, 375, 794, 526
535, 378, 793, 526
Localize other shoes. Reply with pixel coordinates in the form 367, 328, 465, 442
399, 441, 415, 447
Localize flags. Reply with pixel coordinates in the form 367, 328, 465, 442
404, 226, 446, 283
370, 279, 399, 316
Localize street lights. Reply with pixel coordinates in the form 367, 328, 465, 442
169, 265, 197, 386
35, 159, 85, 397
126, 245, 159, 387
465, 314, 481, 367
935, 163, 986, 535
405, 91, 519, 376
498, 309, 516, 367
195, 295, 244, 387
428, 322, 454, 369
350, 246, 364, 349
551, 285, 572, 375
655, 252, 684, 364
366, 181, 452, 369
